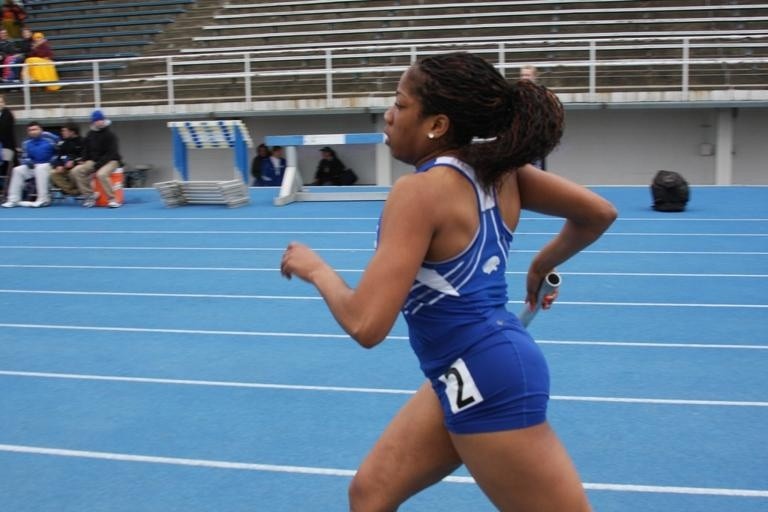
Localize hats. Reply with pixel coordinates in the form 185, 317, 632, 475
91, 109, 103, 122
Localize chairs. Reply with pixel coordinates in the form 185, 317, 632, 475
0, 148, 15, 203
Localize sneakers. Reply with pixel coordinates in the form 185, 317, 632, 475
107, 196, 123, 208
30, 197, 51, 208
82, 192, 99, 208
2, 199, 18, 208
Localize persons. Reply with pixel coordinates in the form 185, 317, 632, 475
518, 64, 546, 170
0, 0, 126, 207
259, 146, 286, 185
278, 52, 619, 512
250, 145, 272, 186
313, 146, 346, 185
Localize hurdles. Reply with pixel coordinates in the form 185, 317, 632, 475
264, 134, 394, 206
155, 120, 254, 207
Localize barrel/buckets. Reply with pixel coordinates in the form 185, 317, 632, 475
126, 168, 147, 188
91, 166, 123, 208
